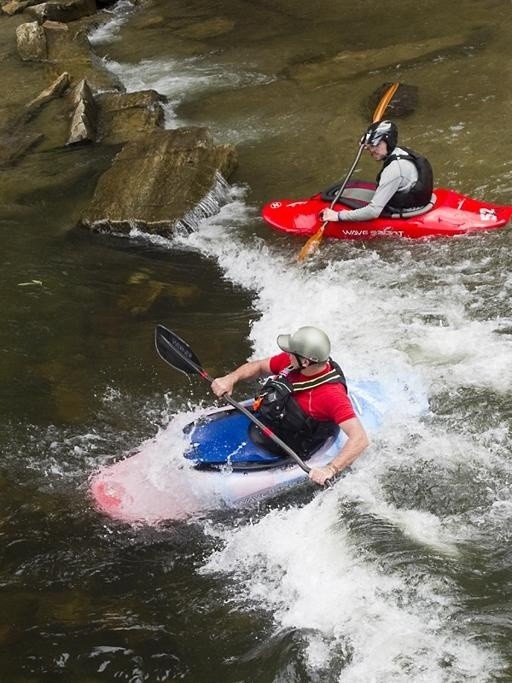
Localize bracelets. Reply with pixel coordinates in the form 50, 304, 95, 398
326, 461, 339, 473
337, 209, 341, 220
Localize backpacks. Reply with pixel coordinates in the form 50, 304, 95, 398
255, 363, 339, 455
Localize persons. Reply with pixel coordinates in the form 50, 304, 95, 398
317, 117, 434, 224
212, 325, 371, 484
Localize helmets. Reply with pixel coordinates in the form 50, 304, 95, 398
361, 120, 398, 154
277, 327, 331, 363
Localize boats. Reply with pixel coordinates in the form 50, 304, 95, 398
262, 177, 512, 242
88, 386, 378, 524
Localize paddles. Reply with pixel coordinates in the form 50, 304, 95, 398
152, 326, 315, 473
296, 85, 400, 257
